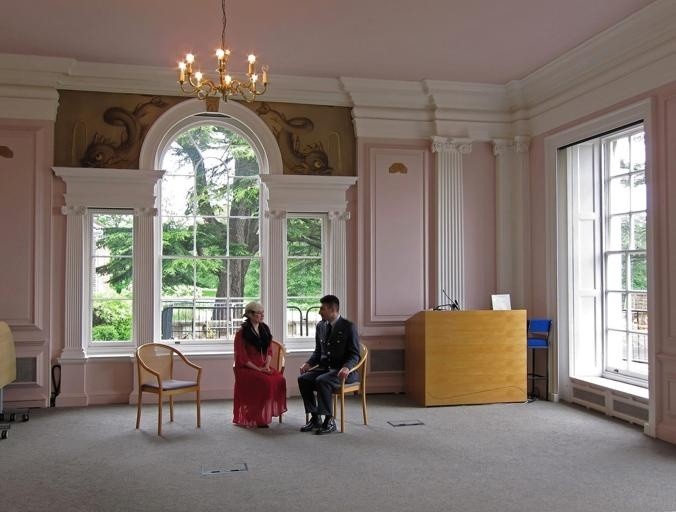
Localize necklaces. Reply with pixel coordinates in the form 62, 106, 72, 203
250, 322, 270, 362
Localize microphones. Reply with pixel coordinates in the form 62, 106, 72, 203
442, 289, 460, 310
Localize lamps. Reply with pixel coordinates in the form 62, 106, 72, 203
175, 1, 270, 104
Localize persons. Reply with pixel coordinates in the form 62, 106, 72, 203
230, 302, 288, 429
297, 295, 361, 435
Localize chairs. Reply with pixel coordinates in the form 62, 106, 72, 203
527, 319, 553, 401
135, 343, 202, 436
232, 339, 286, 423
302, 343, 369, 433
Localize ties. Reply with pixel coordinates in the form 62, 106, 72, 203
325, 323, 331, 339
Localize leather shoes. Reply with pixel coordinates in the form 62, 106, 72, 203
318, 419, 336, 435
300, 418, 321, 432
